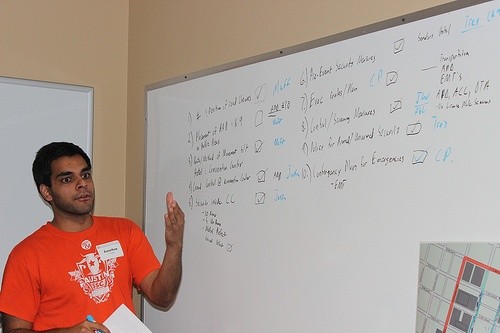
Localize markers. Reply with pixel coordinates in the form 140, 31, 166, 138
86, 314, 102, 333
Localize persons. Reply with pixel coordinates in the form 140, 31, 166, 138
0, 142, 184, 333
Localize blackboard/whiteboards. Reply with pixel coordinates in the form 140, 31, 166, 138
0, 74, 97, 293
138, 0, 500, 333
